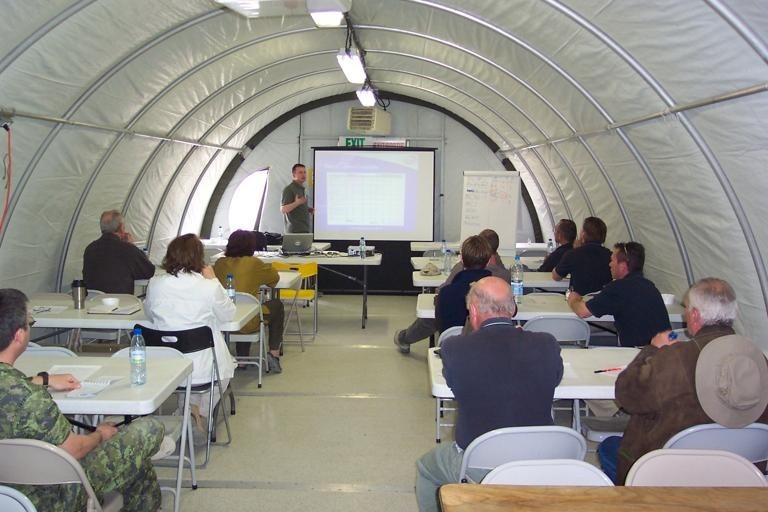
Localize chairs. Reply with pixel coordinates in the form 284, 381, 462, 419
482, 459, 615, 490
1, 483, 41, 512
24, 346, 76, 362
65, 290, 132, 353
670, 328, 691, 341
423, 248, 456, 257
520, 249, 551, 257
271, 261, 319, 335
626, 448, 766, 489
663, 422, 768, 465
436, 326, 465, 347
457, 425, 587, 484
661, 294, 676, 305
2, 438, 108, 512
134, 324, 236, 467
227, 291, 269, 389
115, 344, 199, 510
523, 315, 591, 433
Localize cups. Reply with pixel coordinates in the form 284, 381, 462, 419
70, 280, 88, 309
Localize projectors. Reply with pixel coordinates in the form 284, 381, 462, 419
348, 246, 376, 257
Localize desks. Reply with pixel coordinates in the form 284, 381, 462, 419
412, 271, 571, 296
410, 256, 548, 271
427, 345, 708, 442
211, 250, 384, 329
441, 483, 768, 511
411, 241, 550, 256
12, 354, 197, 512
21, 298, 260, 358
200, 238, 331, 306
416, 291, 685, 337
132, 269, 306, 354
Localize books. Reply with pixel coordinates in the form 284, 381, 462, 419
65, 374, 124, 398
86, 304, 142, 315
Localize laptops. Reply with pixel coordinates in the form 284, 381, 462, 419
282, 233, 314, 254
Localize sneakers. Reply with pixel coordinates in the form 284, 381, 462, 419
394, 328, 410, 353
190, 404, 208, 448
151, 435, 176, 461
237, 359, 249, 370
267, 352, 282, 373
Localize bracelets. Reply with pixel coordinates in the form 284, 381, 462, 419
37, 372, 48, 387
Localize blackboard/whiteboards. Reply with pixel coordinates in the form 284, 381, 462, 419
460, 171, 521, 257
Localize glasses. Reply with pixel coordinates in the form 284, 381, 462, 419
27, 316, 37, 328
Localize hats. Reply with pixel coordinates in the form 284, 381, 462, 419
694, 334, 768, 429
420, 262, 441, 276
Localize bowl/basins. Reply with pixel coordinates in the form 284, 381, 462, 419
102, 298, 119, 307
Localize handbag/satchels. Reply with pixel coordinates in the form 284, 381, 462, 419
263, 233, 283, 245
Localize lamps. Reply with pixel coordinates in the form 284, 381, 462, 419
338, 24, 367, 85
354, 80, 376, 111
311, 5, 343, 31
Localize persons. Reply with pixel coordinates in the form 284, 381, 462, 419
279, 163, 315, 233
551, 217, 614, 298
215, 229, 285, 373
436, 234, 519, 336
0, 288, 177, 512
392, 228, 511, 354
596, 277, 768, 486
524, 218, 577, 296
567, 241, 673, 417
414, 275, 564, 511
81, 209, 156, 296
142, 233, 237, 443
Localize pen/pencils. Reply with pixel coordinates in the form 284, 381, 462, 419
594, 368, 622, 373
112, 307, 118, 311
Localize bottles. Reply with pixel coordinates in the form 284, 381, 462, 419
226, 274, 236, 305
129, 327, 146, 385
142, 248, 149, 255
443, 250, 451, 276
546, 239, 553, 255
510, 256, 524, 304
358, 236, 366, 259
668, 330, 679, 341
440, 240, 446, 267
